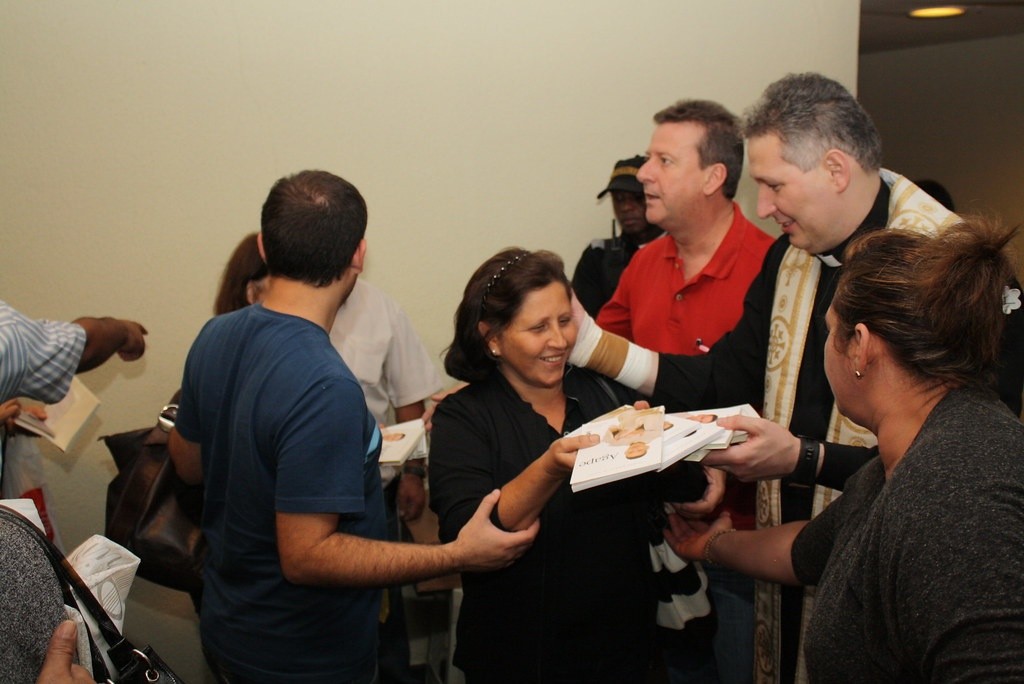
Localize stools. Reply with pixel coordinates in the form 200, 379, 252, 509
425, 588, 465, 684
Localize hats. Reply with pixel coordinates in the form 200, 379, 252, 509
597, 154, 648, 199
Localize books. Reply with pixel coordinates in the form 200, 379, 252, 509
566, 404, 760, 492
14, 376, 100, 454
377, 419, 428, 466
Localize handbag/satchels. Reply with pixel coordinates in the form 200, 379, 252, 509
0, 505, 185, 684
97, 388, 188, 590
584, 367, 721, 654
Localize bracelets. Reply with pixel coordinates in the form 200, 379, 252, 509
704, 528, 737, 566
789, 432, 820, 484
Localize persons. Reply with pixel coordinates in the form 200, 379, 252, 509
430, 246, 656, 684
571, 155, 666, 316
605, 408, 663, 459
663, 218, 1024, 684
381, 429, 405, 447
214, 231, 273, 315
568, 73, 964, 683
330, 281, 446, 683
591, 408, 673, 431
176, 168, 543, 684
423, 100, 776, 683
672, 411, 718, 423
0, 299, 148, 684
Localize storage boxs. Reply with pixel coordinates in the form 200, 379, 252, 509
403, 490, 461, 593
